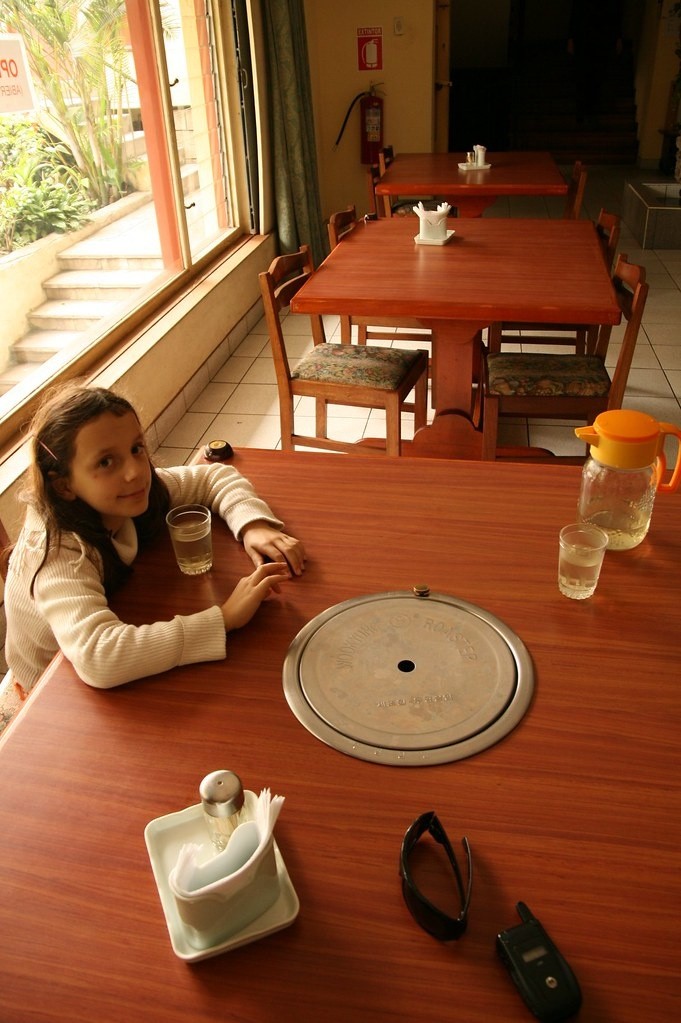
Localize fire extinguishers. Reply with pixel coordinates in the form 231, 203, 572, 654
332, 80, 386, 170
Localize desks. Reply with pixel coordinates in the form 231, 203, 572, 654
373, 152, 568, 218
0, 447, 681, 1023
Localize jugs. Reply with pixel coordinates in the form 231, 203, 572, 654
575, 410, 681, 550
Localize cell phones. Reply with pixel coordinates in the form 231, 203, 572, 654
496, 901, 582, 1023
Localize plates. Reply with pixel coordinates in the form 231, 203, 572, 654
144, 788, 300, 963
414, 230, 455, 246
457, 162, 491, 170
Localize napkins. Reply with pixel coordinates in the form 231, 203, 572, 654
171, 788, 285, 950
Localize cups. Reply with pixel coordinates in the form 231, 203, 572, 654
165, 504, 213, 574
558, 525, 608, 599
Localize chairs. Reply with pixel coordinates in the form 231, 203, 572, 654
366, 166, 386, 221
378, 145, 434, 205
475, 255, 650, 462
564, 160, 589, 218
259, 245, 429, 459
328, 203, 433, 352
489, 208, 622, 358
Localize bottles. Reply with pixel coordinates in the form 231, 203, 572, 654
466, 152, 474, 166
200, 771, 247, 852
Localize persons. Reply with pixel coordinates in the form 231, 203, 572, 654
5, 384, 308, 700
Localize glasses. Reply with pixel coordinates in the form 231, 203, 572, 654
398, 810, 473, 943
